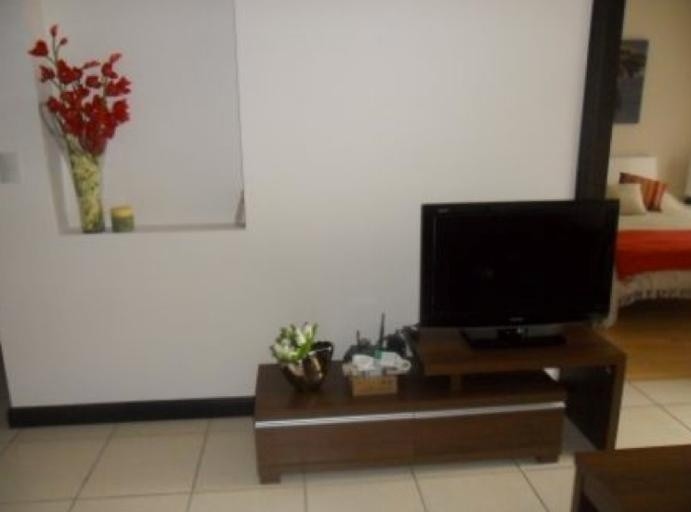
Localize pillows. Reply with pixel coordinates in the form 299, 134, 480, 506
613, 172, 668, 214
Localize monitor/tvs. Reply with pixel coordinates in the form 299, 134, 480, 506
420, 198, 619, 350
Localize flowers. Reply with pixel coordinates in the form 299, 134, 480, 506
27, 24, 131, 155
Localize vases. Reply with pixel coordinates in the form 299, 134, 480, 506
64, 151, 106, 232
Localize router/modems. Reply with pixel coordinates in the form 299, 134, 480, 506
344, 345, 388, 364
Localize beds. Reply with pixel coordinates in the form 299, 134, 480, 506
607, 155, 690, 318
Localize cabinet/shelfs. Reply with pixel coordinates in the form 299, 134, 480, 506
251, 325, 629, 483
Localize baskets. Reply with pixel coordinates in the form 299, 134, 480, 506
348, 376, 397, 397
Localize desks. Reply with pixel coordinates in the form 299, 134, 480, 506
569, 445, 691, 512
